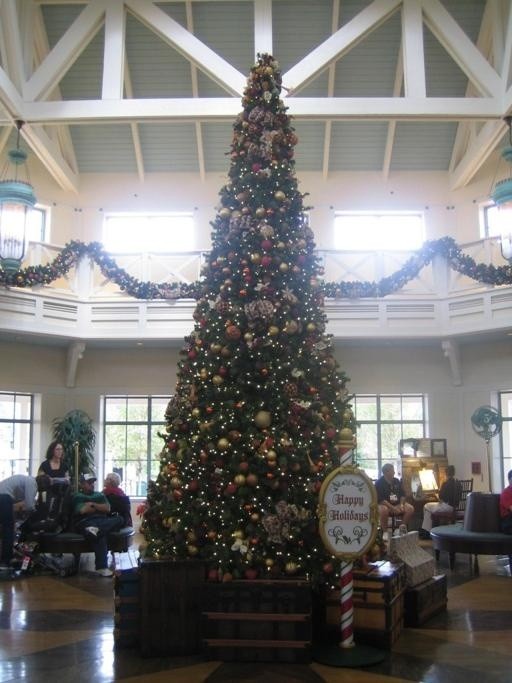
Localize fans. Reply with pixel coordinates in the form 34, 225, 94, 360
471, 405, 503, 445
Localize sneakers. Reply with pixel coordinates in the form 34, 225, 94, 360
95, 568, 113, 577
85, 526, 99, 541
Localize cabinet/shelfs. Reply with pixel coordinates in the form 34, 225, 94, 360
402, 457, 448, 496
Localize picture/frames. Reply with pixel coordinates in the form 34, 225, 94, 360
400, 439, 417, 458
431, 439, 447, 457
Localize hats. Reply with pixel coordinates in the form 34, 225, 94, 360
81, 473, 96, 481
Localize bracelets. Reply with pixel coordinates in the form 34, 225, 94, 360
91, 501, 95, 509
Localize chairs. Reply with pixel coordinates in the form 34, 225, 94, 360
456, 478, 473, 513
23, 492, 136, 577
429, 491, 512, 580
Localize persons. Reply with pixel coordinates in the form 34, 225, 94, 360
375, 463, 415, 541
38, 440, 71, 515
73, 473, 125, 577
0, 474, 53, 570
102, 472, 133, 526
418, 464, 461, 540
499, 469, 512, 536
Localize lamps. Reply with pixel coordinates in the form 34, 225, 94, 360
488, 115, 512, 268
0, 119, 37, 284
